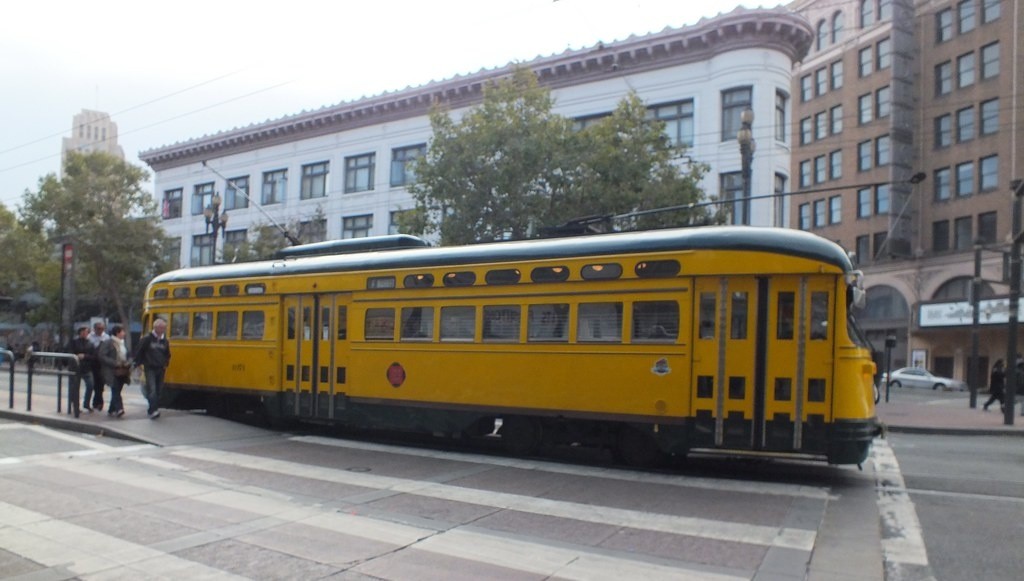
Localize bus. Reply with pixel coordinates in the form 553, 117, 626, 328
143, 224, 896, 471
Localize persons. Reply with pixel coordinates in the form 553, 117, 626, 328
61, 327, 95, 412
406, 315, 429, 337
1016, 359, 1024, 416
88, 322, 110, 409
134, 319, 171, 418
984, 359, 1006, 413
97, 325, 129, 416
24, 341, 63, 370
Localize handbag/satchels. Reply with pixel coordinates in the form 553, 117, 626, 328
116, 366, 131, 383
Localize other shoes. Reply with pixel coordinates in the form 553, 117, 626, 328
85, 405, 93, 412
116, 409, 124, 418
149, 410, 160, 419
107, 412, 115, 417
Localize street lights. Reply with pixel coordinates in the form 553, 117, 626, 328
203, 191, 228, 265
736, 102, 757, 227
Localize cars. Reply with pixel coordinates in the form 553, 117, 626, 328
883, 367, 965, 393
